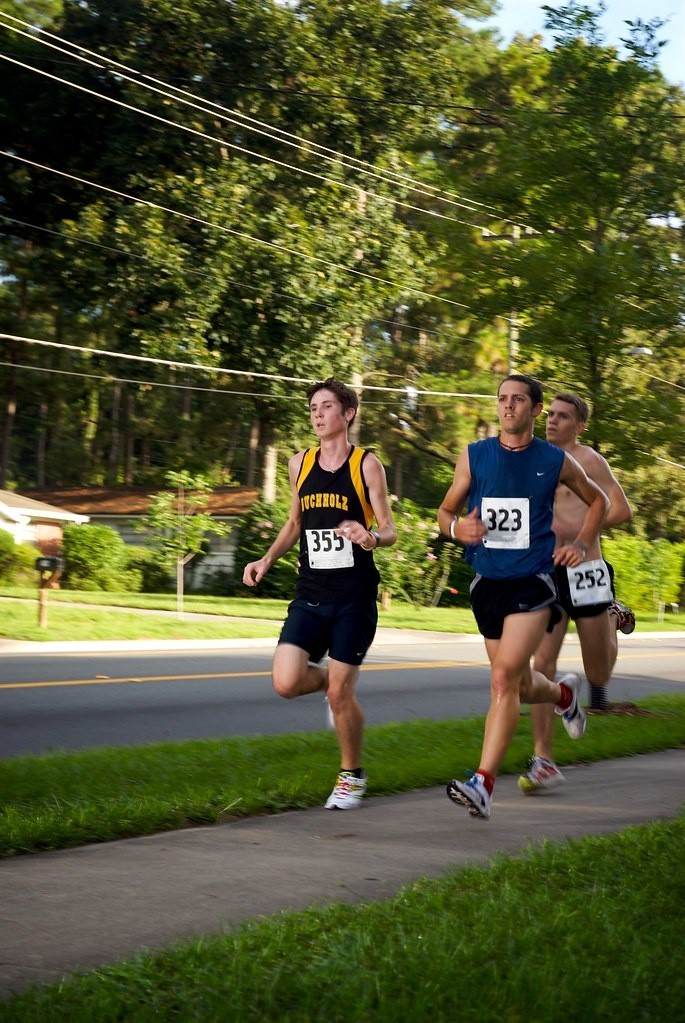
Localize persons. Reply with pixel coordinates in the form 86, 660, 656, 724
519, 392, 635, 795
243, 381, 398, 810
437, 376, 611, 820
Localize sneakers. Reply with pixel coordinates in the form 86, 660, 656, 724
517, 754, 566, 794
609, 599, 636, 635
325, 768, 368, 810
446, 769, 491, 819
323, 665, 359, 727
551, 672, 586, 739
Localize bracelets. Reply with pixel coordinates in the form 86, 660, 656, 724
572, 541, 587, 554
450, 520, 457, 541
360, 530, 381, 550
